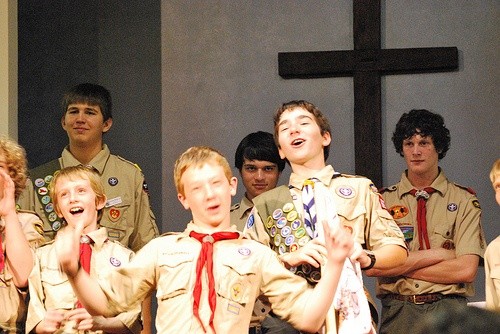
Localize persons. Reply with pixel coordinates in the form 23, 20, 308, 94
25, 166, 143, 334
14, 82, 160, 253
54, 145, 355, 334
229, 130, 301, 334
0, 135, 46, 334
241, 99, 411, 334
374, 108, 488, 334
483, 158, 500, 311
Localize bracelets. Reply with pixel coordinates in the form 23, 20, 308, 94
72, 261, 81, 278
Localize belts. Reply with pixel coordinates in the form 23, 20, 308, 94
386, 293, 459, 304
249, 326, 270, 334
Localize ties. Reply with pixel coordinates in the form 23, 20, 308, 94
76, 235, 109, 325
189, 230, 247, 334
409, 187, 435, 250
288, 172, 341, 240
0, 234, 5, 272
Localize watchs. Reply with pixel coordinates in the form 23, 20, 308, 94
361, 249, 376, 270
